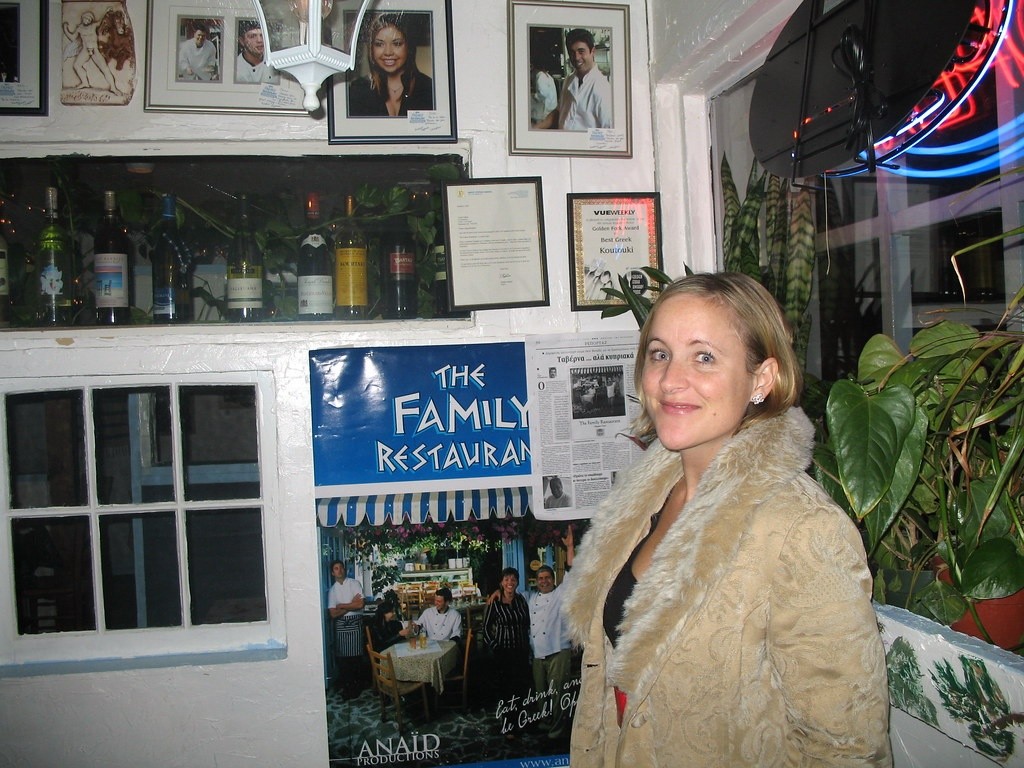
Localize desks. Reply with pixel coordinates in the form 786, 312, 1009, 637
379, 637, 458, 712
443, 601, 488, 633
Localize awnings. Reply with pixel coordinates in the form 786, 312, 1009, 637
313, 488, 535, 526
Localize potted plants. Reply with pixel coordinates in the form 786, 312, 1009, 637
812, 167, 1024, 648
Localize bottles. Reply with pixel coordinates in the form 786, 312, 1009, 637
335, 194, 368, 321
35, 185, 72, 327
380, 183, 417, 319
225, 192, 263, 323
0, 229, 11, 328
151, 191, 192, 324
93, 189, 130, 325
296, 192, 334, 321
432, 221, 465, 319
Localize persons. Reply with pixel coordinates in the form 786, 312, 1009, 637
549, 368, 556, 378
572, 376, 616, 414
349, 12, 432, 117
487, 524, 576, 737
530, 62, 557, 129
178, 21, 216, 81
557, 29, 614, 130
480, 567, 536, 731
409, 588, 464, 647
237, 20, 279, 83
369, 600, 412, 702
327, 561, 365, 695
545, 477, 572, 509
561, 273, 893, 768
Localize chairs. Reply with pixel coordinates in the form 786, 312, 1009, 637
364, 588, 472, 737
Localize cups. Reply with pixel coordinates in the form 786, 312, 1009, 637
410, 637, 416, 650
420, 628, 426, 648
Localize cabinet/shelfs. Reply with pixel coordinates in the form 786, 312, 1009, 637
399, 566, 473, 589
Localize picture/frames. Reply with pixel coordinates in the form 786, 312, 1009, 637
321, 0, 457, 144
507, 1, 632, 158
144, 0, 316, 115
0, 1, 50, 116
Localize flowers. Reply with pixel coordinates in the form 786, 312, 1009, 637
334, 518, 593, 566
